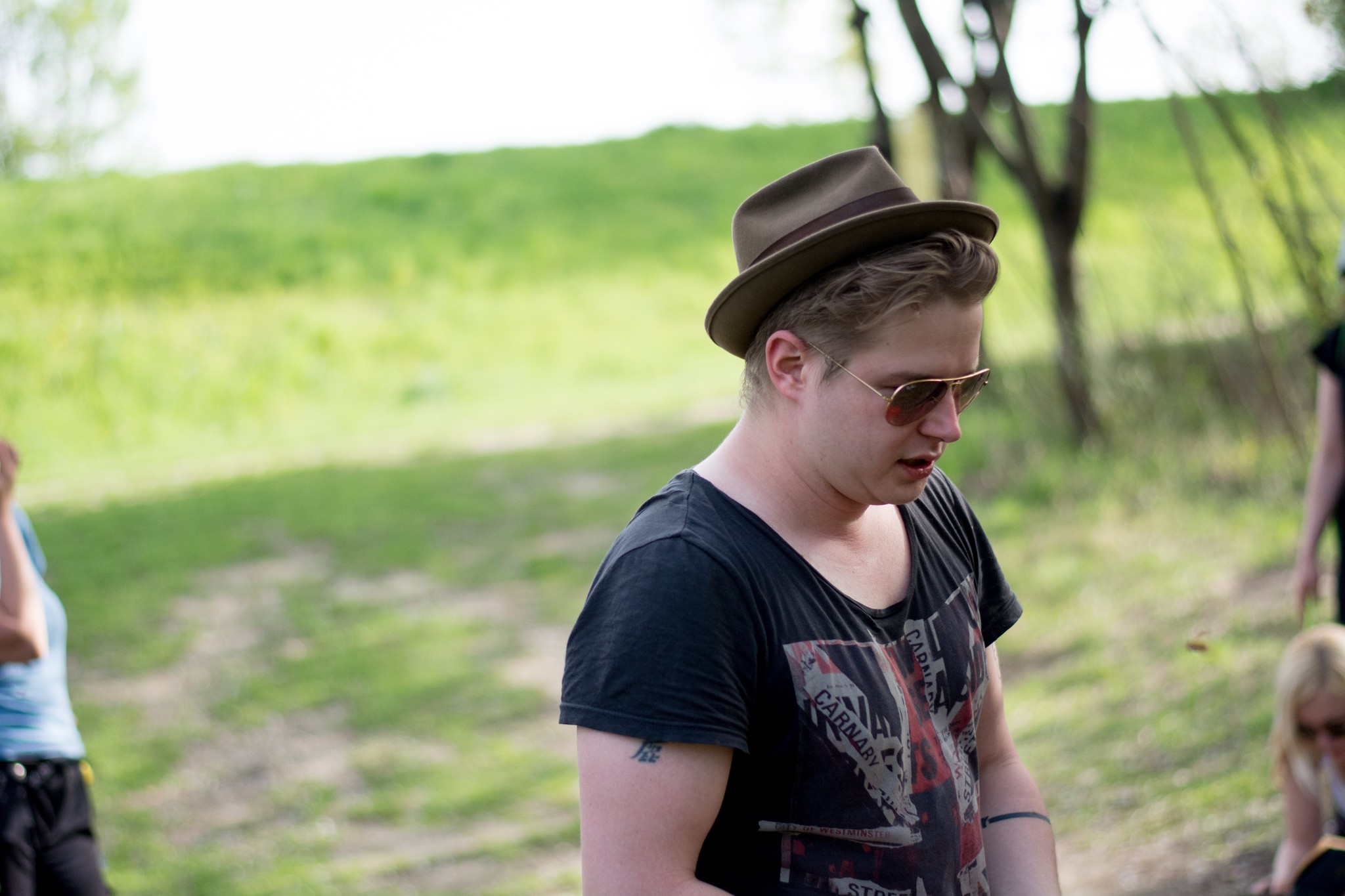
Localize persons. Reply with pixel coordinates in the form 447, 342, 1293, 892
1283, 241, 1345, 624
559, 143, 1064, 896
0, 436, 115, 896
1273, 621, 1345, 896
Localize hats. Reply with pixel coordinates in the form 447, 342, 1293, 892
706, 144, 1000, 359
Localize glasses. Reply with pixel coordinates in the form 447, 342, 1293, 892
803, 340, 990, 428
1299, 722, 1344, 741
